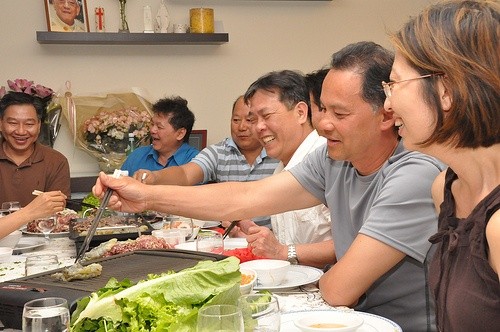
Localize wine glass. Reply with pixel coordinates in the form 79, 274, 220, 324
35, 214, 57, 243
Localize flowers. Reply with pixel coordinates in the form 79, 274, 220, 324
78, 105, 156, 156
0, 77, 54, 107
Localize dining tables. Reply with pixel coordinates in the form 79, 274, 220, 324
0, 196, 356, 332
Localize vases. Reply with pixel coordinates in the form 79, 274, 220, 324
37, 109, 53, 147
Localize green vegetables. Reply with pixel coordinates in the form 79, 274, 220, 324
79, 193, 114, 216
69, 256, 273, 332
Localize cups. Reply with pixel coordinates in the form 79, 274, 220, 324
141, 208, 158, 223
236, 293, 281, 332
195, 304, 244, 332
173, 24, 187, 33
21, 297, 70, 332
152, 214, 193, 247
25, 254, 60, 276
0, 201, 21, 217
195, 232, 224, 255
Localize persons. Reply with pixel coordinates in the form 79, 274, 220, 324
0, 91, 71, 210
0, 191, 68, 241
49, 0, 86, 32
93, 0, 500, 332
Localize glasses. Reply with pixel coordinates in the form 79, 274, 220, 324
382, 73, 446, 99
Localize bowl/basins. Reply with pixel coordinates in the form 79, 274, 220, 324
240, 260, 291, 287
0, 230, 23, 249
0, 247, 13, 264
293, 310, 364, 332
239, 268, 257, 295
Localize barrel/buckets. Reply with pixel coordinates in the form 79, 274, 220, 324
190, 8, 214, 33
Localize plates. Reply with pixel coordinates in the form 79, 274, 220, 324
175, 237, 248, 251
14, 237, 47, 253
21, 227, 70, 236
253, 264, 324, 290
150, 220, 221, 230
196, 230, 222, 239
279, 309, 403, 332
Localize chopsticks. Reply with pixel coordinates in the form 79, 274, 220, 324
223, 220, 240, 239
32, 189, 98, 210
74, 169, 122, 263
124, 172, 147, 225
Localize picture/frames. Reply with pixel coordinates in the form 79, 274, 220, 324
182, 129, 208, 152
44, 0, 91, 33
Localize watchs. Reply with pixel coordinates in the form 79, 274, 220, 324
286, 242, 300, 265
